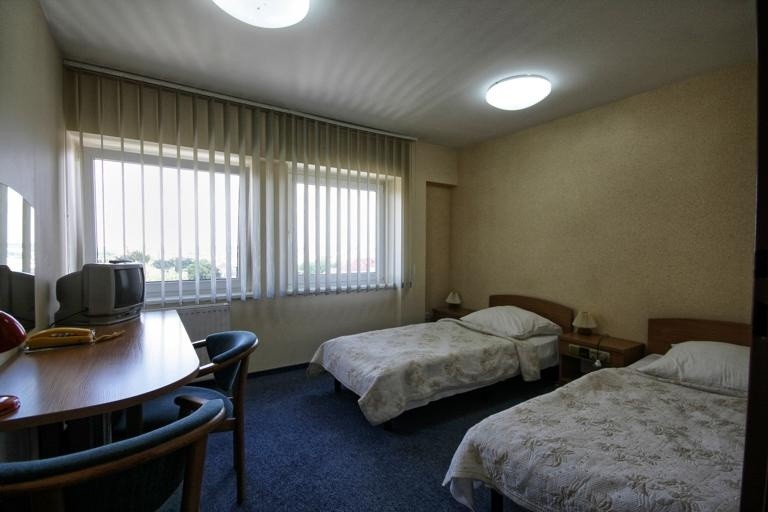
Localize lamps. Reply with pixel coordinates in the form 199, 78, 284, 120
572, 311, 597, 336
486, 74, 552, 111
447, 288, 460, 310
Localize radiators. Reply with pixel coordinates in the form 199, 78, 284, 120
175, 304, 233, 365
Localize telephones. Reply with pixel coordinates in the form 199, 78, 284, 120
25, 328, 125, 346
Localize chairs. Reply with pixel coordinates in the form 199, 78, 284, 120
189, 330, 258, 510
0, 393, 223, 512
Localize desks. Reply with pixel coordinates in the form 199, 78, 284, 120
0, 308, 201, 459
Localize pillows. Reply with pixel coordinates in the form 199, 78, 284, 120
459, 305, 564, 340
638, 340, 752, 399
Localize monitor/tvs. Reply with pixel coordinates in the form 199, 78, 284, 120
0, 265, 35, 333
54, 263, 145, 326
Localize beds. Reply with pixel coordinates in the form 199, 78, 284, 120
306, 296, 573, 426
440, 318, 753, 511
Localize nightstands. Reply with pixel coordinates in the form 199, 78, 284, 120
557, 331, 645, 385
432, 304, 476, 321
